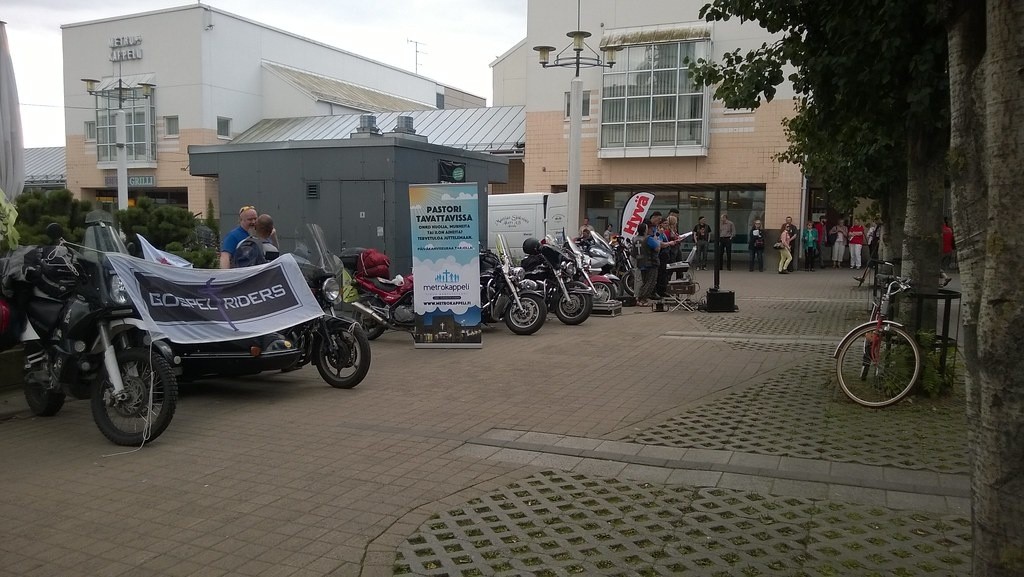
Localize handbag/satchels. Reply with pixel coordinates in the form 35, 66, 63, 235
773, 242, 785, 250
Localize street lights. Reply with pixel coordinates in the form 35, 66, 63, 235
81, 48, 157, 241
531, 0, 625, 239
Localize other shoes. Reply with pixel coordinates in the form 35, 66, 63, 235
779, 268, 789, 274
719, 268, 723, 270
726, 267, 731, 271
825, 226, 838, 247
803, 264, 861, 272
749, 269, 753, 272
696, 267, 700, 271
789, 267, 792, 272
649, 293, 663, 300
636, 301, 648, 307
759, 268, 763, 272
658, 291, 673, 297
702, 267, 708, 270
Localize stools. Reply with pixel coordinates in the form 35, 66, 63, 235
664, 292, 695, 313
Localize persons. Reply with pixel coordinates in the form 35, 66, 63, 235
829, 218, 848, 268
941, 221, 953, 286
580, 218, 595, 235
778, 217, 798, 274
219, 206, 280, 269
749, 217, 767, 272
634, 208, 683, 306
692, 216, 711, 271
803, 221, 818, 272
234, 214, 279, 263
848, 218, 864, 269
865, 219, 881, 268
720, 214, 736, 270
604, 223, 612, 243
813, 216, 828, 269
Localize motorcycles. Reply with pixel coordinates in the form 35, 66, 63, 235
523, 227, 632, 326
479, 235, 547, 335
341, 241, 413, 342
19, 222, 178, 447
150, 223, 371, 389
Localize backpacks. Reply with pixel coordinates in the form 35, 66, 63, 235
232, 236, 270, 268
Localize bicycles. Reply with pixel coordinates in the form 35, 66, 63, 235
834, 259, 922, 409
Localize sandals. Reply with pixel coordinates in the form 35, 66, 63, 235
938, 283, 943, 287
943, 276, 952, 286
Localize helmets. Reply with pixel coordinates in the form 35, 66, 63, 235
523, 238, 543, 255
20, 248, 83, 299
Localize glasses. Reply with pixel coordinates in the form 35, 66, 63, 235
239, 206, 255, 216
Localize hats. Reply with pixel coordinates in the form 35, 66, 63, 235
667, 208, 680, 214
649, 211, 662, 218
699, 216, 705, 221
